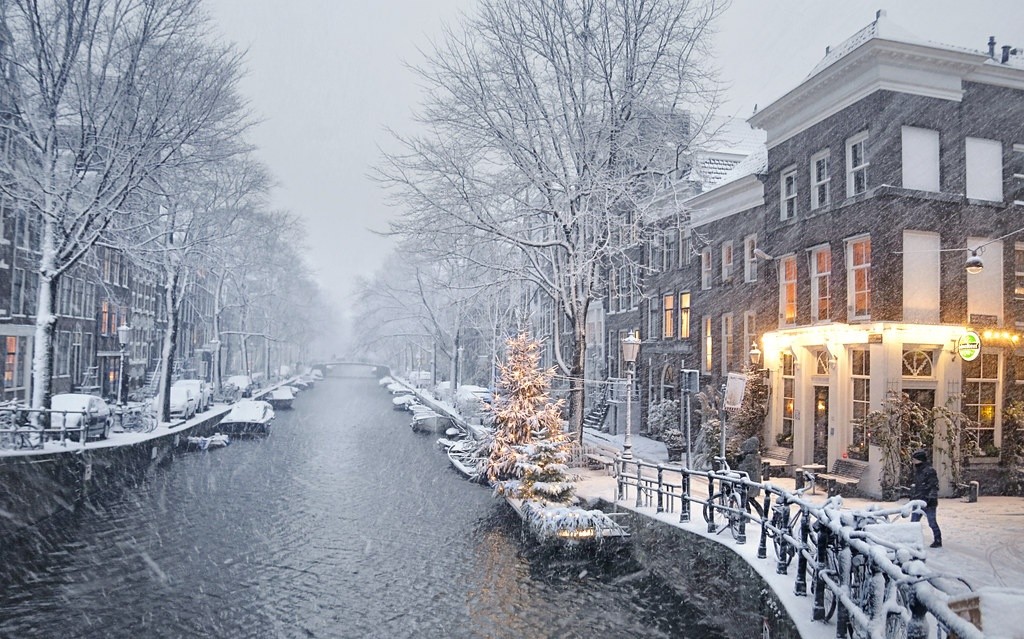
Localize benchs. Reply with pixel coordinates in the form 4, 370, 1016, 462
760, 446, 793, 478
817, 459, 869, 497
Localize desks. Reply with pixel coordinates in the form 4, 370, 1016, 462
802, 465, 826, 494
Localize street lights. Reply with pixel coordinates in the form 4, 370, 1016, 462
620, 330, 641, 459
110, 321, 131, 433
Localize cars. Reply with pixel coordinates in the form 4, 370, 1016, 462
0, 366, 323, 450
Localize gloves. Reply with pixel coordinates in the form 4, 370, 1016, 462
928, 498, 933, 507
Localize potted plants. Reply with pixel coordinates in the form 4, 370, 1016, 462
663, 428, 686, 461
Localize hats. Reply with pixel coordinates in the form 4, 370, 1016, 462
913, 452, 928, 462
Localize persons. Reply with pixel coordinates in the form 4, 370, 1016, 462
910, 451, 942, 548
123, 374, 130, 405
739, 437, 764, 523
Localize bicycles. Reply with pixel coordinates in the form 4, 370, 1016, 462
704, 456, 975, 639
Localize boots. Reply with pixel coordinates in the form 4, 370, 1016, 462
930, 534, 942, 548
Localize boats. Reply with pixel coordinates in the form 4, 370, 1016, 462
380, 376, 631, 547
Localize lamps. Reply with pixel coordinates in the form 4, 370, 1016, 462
749, 341, 769, 378
